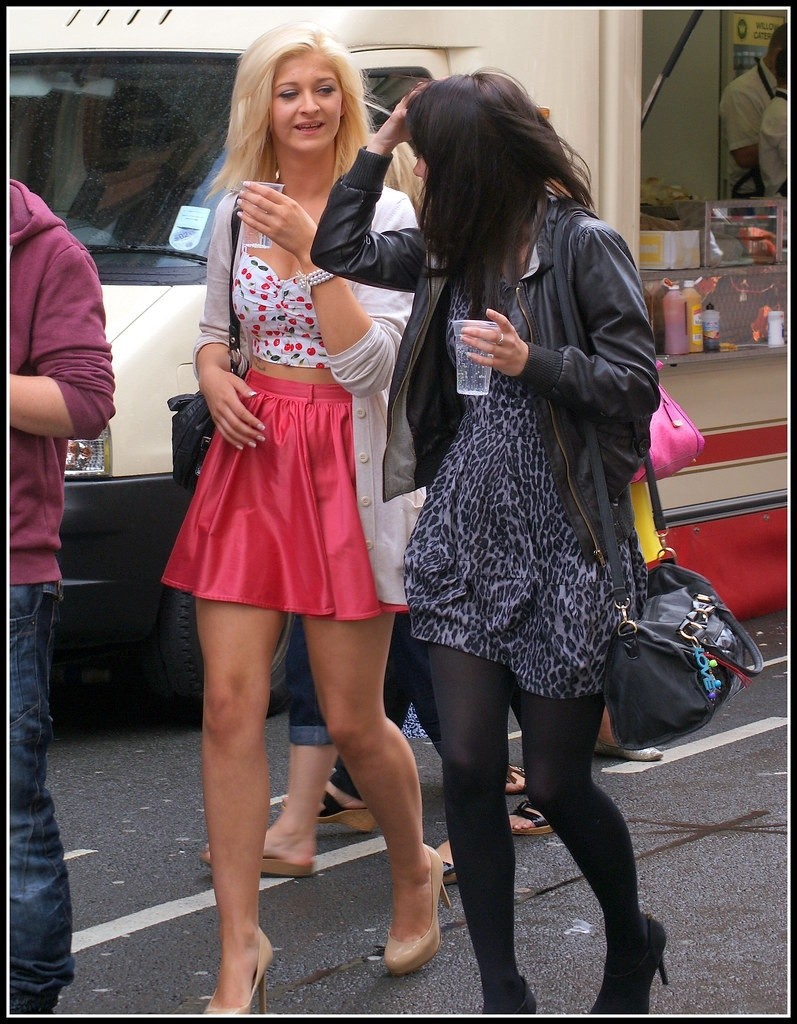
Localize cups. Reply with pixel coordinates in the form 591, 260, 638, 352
767, 311, 785, 348
451, 319, 497, 396
240, 180, 285, 249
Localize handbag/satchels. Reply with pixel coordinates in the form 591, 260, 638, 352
600, 558, 765, 748
623, 360, 705, 484
166, 387, 217, 493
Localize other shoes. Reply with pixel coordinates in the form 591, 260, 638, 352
504, 763, 527, 795
10, 984, 60, 1014
594, 739, 662, 762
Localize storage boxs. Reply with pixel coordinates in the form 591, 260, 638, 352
640, 228, 700, 270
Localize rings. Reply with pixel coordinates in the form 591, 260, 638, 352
496, 333, 504, 345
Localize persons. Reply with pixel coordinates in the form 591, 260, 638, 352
198, 129, 664, 885
160, 18, 449, 1015
10, 177, 118, 1014
718, 24, 787, 215
757, 48, 788, 198
310, 68, 668, 1014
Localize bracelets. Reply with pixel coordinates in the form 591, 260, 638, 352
297, 267, 336, 294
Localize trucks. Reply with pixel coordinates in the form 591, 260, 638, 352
5, 4, 788, 735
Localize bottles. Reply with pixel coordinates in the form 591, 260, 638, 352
661, 279, 690, 356
682, 277, 703, 353
702, 302, 720, 353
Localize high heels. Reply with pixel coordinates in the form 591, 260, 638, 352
385, 845, 453, 975
589, 915, 670, 1015
280, 788, 376, 833
479, 974, 538, 1015
203, 927, 273, 1017
507, 799, 555, 834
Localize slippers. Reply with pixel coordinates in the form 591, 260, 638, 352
201, 830, 312, 877
433, 838, 457, 884
